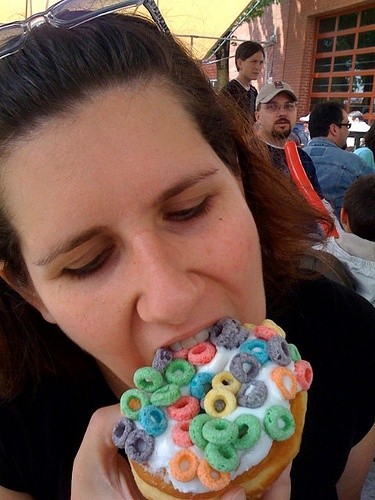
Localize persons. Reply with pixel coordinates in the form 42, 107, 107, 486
300, 101, 375, 223
0, 0, 375, 500
250, 81, 336, 247
220, 40, 265, 133
353, 122, 375, 169
346, 109, 371, 145
310, 175, 375, 307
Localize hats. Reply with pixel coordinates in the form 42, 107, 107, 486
255, 81, 297, 111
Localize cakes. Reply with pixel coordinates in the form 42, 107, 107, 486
111, 316, 315, 500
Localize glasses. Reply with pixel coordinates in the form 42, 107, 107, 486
265, 101, 296, 111
335, 123, 351, 129
0, 0, 174, 58
